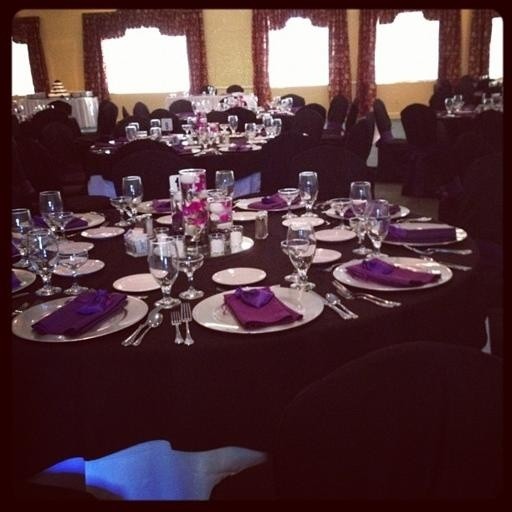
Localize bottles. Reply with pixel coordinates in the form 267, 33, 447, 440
255, 211, 268, 240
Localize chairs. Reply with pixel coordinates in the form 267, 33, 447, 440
12, 76, 502, 211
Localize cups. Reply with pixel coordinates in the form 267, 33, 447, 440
146, 226, 205, 309
168, 168, 244, 254
11, 100, 56, 123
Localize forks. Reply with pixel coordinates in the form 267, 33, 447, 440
331, 280, 400, 308
403, 245, 472, 255
179, 300, 196, 347
170, 311, 185, 346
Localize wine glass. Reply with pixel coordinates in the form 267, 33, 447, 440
287, 218, 317, 292
328, 180, 389, 258
281, 239, 311, 283
11, 190, 89, 297
278, 188, 299, 219
109, 177, 153, 257
444, 93, 502, 118
298, 171, 319, 218
126, 82, 294, 156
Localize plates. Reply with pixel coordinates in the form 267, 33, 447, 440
55, 258, 105, 277
56, 212, 106, 233
334, 256, 454, 292
211, 266, 267, 285
113, 272, 161, 291
12, 293, 148, 341
381, 222, 467, 247
325, 204, 411, 220
237, 196, 305, 212
283, 218, 324, 228
311, 247, 342, 264
232, 210, 256, 221
139, 199, 172, 214
157, 214, 172, 226
313, 229, 356, 243
193, 287, 323, 336
80, 227, 126, 240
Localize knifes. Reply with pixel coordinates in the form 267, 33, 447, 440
313, 291, 352, 320
122, 305, 162, 347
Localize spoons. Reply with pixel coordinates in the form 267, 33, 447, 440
327, 292, 358, 319
134, 312, 163, 346
